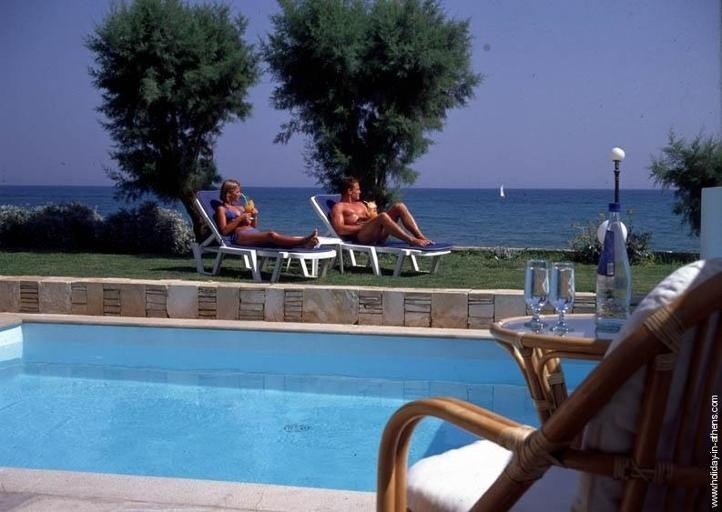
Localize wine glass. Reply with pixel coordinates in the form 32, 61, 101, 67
244, 200, 256, 221
524, 259, 578, 335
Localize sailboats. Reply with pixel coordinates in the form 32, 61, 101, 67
500, 184, 505, 197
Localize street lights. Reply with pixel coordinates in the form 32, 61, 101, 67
609, 147, 627, 204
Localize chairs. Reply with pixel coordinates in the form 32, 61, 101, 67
376, 257, 720, 511
185, 184, 455, 280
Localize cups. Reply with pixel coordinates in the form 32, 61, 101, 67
368, 201, 379, 218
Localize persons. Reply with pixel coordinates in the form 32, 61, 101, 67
330, 175, 436, 247
217, 178, 320, 249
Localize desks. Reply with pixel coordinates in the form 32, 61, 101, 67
482, 306, 609, 418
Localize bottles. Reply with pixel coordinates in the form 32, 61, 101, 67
594, 201, 632, 332
